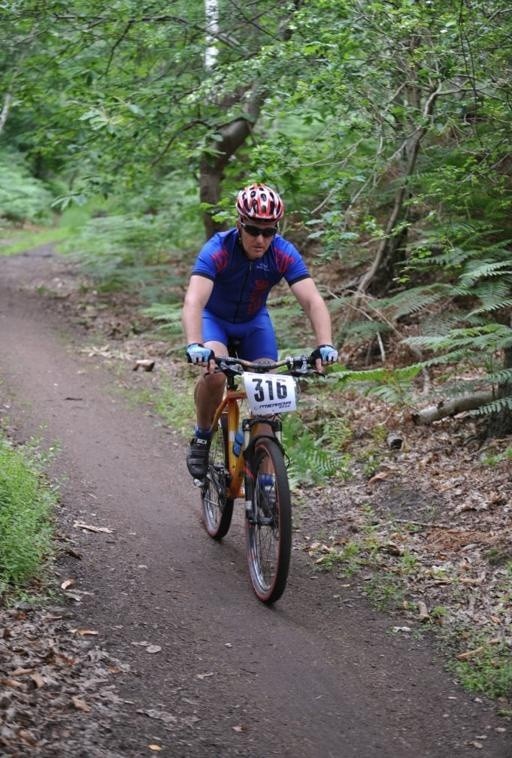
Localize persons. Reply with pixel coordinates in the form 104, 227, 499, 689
178, 181, 339, 533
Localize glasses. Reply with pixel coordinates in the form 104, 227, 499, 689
239, 222, 278, 237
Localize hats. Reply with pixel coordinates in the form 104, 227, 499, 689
236, 183, 285, 226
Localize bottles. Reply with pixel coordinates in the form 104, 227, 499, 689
232, 418, 248, 459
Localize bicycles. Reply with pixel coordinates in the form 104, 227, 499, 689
186, 352, 327, 604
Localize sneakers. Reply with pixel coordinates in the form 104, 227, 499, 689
257, 485, 277, 522
187, 438, 210, 479
